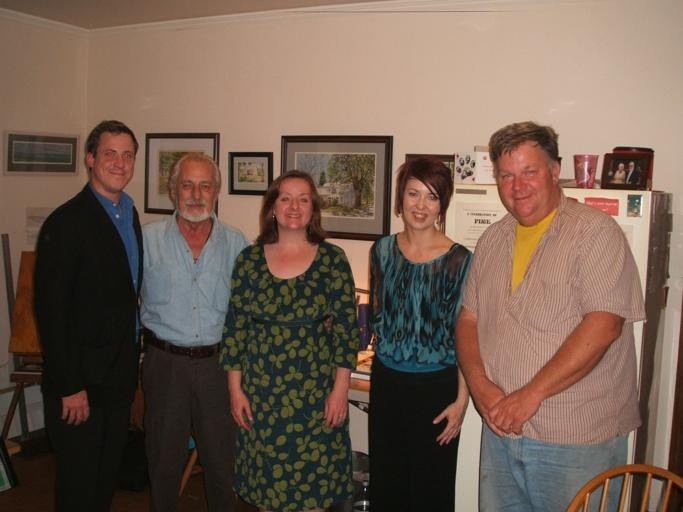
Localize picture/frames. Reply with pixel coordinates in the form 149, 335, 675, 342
279, 134, 394, 244
3, 129, 79, 176
227, 151, 274, 196
143, 132, 219, 220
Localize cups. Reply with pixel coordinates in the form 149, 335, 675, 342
573, 152, 598, 186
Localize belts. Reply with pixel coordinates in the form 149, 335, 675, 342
149, 335, 221, 358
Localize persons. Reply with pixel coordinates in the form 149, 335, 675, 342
30, 120, 145, 509
612, 162, 625, 184
454, 121, 646, 512
217, 170, 360, 511
363, 157, 473, 511
136, 151, 335, 510
624, 161, 640, 184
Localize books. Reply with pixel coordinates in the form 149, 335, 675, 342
350, 371, 370, 381
356, 358, 373, 373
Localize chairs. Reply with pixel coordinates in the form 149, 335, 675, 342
562, 463, 682, 512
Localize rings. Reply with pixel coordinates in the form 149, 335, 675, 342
338, 414, 344, 419
